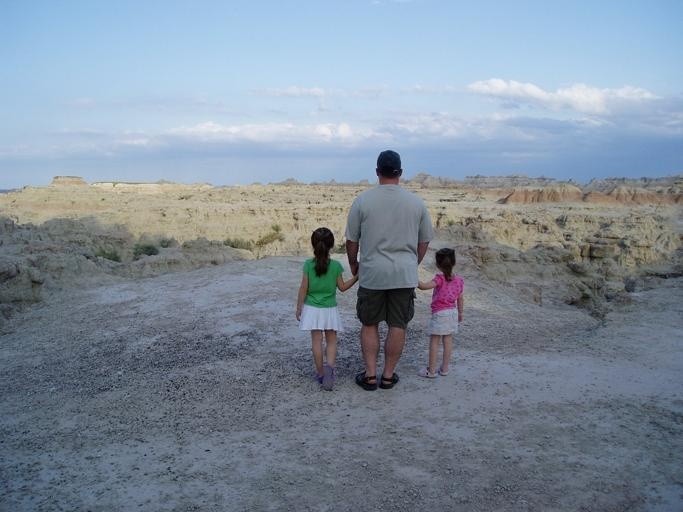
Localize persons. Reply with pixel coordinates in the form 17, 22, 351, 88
345, 149, 436, 390
417, 248, 463, 378
296, 226, 358, 391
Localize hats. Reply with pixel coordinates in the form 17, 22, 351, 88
374, 150, 402, 177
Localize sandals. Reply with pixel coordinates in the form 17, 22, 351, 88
355, 370, 379, 392
315, 372, 326, 385
378, 373, 399, 390
321, 365, 336, 391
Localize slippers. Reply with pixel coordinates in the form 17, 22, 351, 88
437, 365, 450, 376
416, 366, 438, 378
